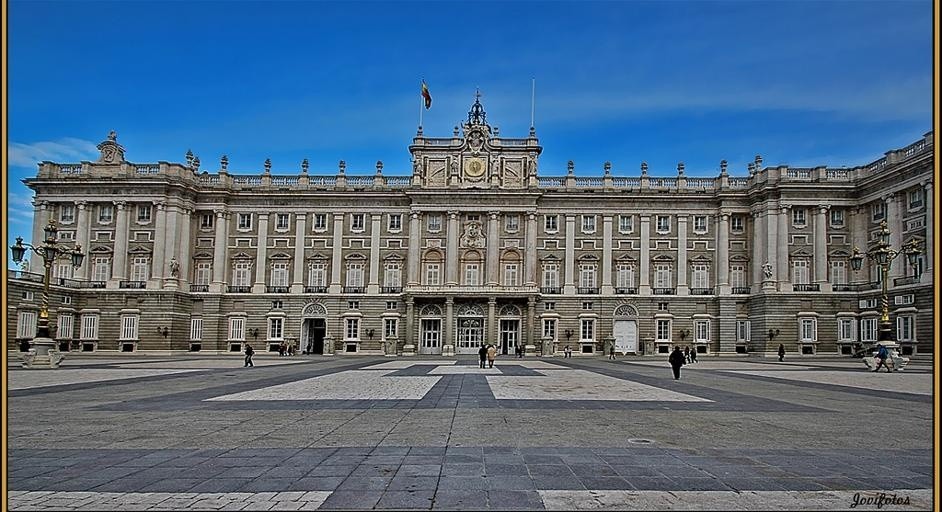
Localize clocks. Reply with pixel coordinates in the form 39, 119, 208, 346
464, 158, 486, 176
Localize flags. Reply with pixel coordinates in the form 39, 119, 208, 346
422, 79, 432, 110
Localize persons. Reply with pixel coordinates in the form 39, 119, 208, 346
669, 346, 686, 379
478, 344, 497, 368
243, 344, 254, 367
874, 344, 889, 373
515, 345, 525, 358
762, 260, 772, 279
778, 343, 785, 362
307, 344, 311, 355
685, 346, 698, 364
564, 346, 572, 358
609, 346, 616, 359
169, 256, 180, 279
20, 338, 30, 367
278, 342, 295, 356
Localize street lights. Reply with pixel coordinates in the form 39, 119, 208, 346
851, 221, 921, 341
10, 219, 86, 336
